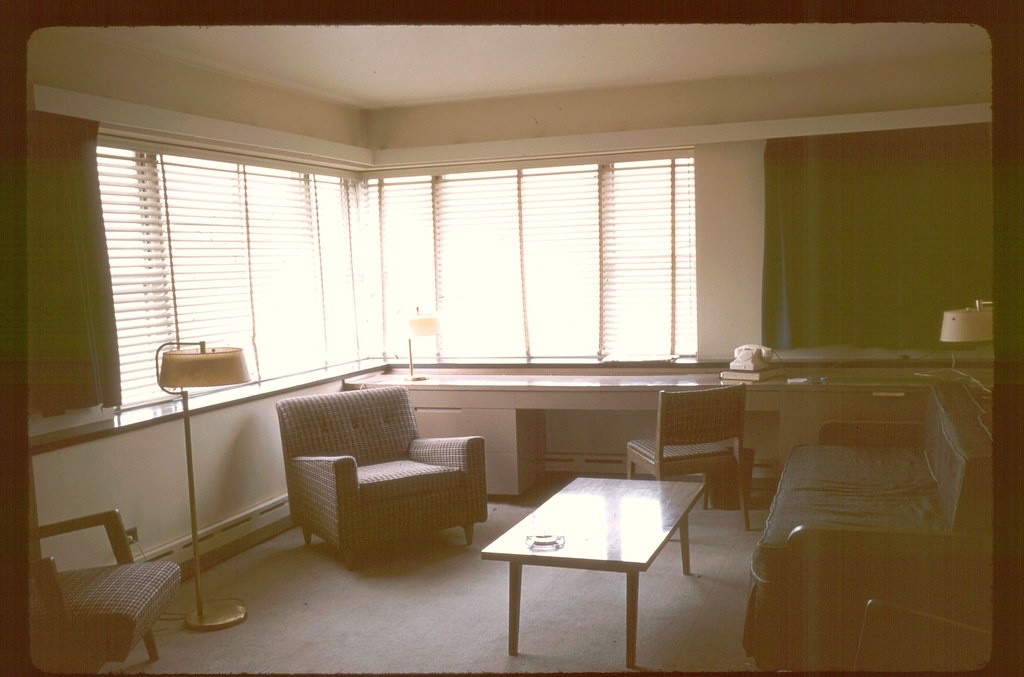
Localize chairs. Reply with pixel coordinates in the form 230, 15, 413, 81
30, 455, 185, 677
627, 384, 753, 535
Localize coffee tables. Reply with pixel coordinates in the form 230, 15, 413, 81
481, 477, 708, 668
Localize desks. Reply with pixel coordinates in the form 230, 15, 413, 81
347, 363, 996, 502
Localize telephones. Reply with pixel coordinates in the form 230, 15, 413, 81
730, 343, 772, 371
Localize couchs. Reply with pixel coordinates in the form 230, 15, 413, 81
274, 384, 489, 574
740, 381, 993, 671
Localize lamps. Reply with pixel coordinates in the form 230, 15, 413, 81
940, 298, 995, 355
402, 303, 441, 382
154, 338, 253, 631
